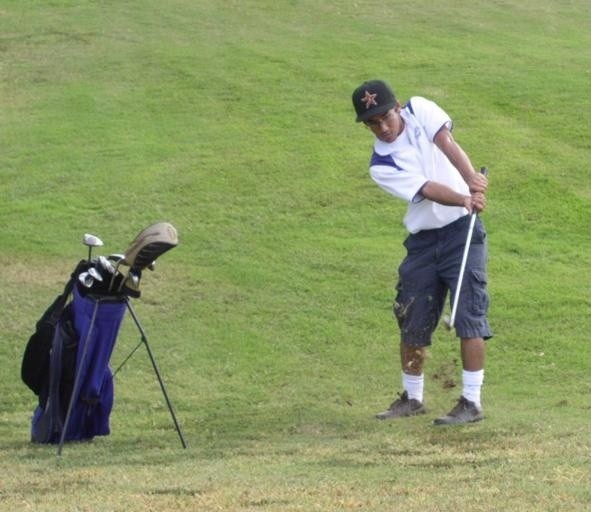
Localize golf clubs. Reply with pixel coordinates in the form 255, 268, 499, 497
442, 167, 486, 332
79, 223, 180, 296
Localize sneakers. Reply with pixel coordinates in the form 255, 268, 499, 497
376, 390, 429, 421
432, 395, 485, 426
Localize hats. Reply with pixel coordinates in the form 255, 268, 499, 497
349, 79, 400, 124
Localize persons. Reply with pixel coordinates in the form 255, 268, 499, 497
351, 79, 494, 428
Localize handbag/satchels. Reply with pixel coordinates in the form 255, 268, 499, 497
21, 256, 82, 397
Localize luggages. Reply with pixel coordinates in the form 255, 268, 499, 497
30, 259, 130, 444
